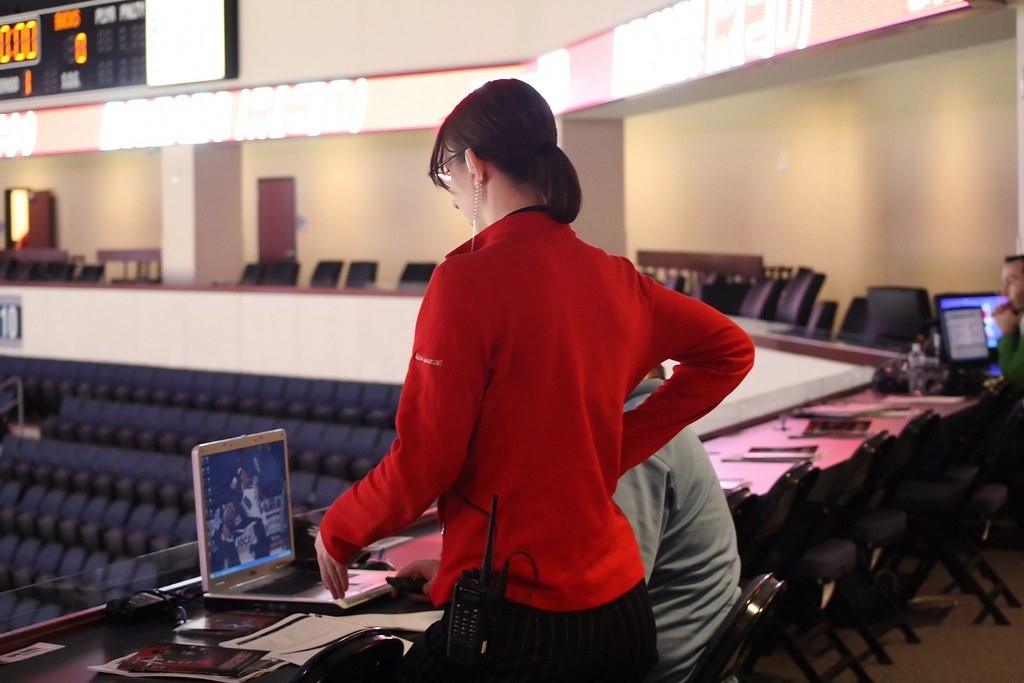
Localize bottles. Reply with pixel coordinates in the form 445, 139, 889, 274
907, 343, 925, 398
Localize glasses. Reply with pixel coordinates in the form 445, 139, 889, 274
428, 146, 475, 188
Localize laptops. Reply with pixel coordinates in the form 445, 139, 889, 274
191, 429, 398, 611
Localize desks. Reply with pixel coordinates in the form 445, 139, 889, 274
0, 355, 1005, 683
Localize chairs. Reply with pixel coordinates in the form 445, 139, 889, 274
0, 258, 1024, 683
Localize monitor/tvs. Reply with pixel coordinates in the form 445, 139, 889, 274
934, 290, 1012, 351
939, 306, 989, 365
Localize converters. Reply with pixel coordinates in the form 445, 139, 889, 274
104, 590, 176, 622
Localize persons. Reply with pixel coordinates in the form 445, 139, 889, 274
390, 364, 743, 683
314, 78, 755, 683
211, 457, 291, 574
983, 255, 1024, 387
